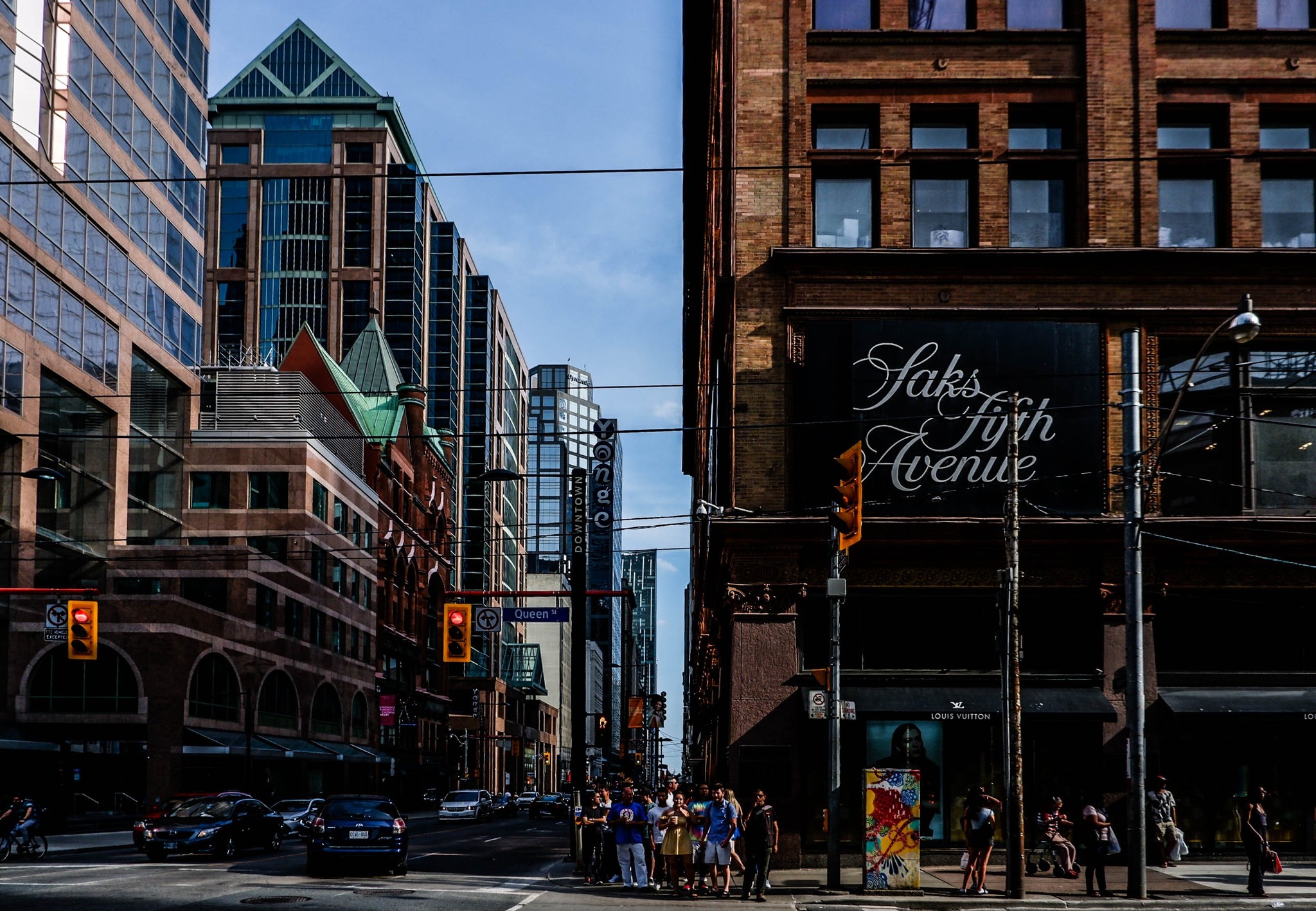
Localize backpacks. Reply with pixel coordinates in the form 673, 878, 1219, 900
22, 799, 38, 813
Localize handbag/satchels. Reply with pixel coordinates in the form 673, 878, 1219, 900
960, 852, 970, 870
1106, 826, 1121, 854
1265, 850, 1282, 874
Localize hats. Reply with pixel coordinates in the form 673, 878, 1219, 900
1258, 790, 1266, 793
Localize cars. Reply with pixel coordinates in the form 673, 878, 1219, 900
490, 792, 571, 821
438, 789, 495, 827
142, 796, 285, 863
132, 792, 253, 854
269, 799, 325, 834
307, 793, 409, 876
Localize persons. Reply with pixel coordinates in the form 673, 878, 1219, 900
1082, 791, 1114, 898
1148, 775, 1179, 868
959, 787, 1002, 895
1043, 794, 1079, 880
1242, 787, 1273, 898
0, 795, 39, 848
580, 774, 779, 901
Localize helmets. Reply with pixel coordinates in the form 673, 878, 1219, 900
1157, 776, 1168, 783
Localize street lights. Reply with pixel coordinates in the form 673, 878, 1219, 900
1120, 291, 1262, 899
477, 468, 586, 862
479, 701, 511, 790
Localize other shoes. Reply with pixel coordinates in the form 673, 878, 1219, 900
583, 877, 593, 886
689, 889, 698, 897
682, 882, 695, 890
756, 893, 766, 902
671, 889, 680, 897
698, 880, 709, 890
648, 877, 658, 886
765, 879, 771, 888
608, 874, 619, 883
977, 888, 990, 895
954, 888, 967, 895
1065, 870, 1080, 880
595, 877, 603, 885
1249, 891, 1271, 898
742, 891, 750, 898
735, 870, 745, 877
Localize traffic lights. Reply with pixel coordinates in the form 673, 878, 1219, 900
635, 753, 642, 765
67, 600, 98, 660
443, 604, 471, 663
828, 440, 864, 551
544, 752, 550, 765
595, 715, 609, 748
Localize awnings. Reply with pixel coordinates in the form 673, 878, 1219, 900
800, 686, 1119, 722
1156, 686, 1316, 714
0, 726, 397, 764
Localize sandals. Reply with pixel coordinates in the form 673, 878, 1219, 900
704, 889, 720, 896
721, 890, 731, 898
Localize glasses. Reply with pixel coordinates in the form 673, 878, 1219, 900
755, 794, 763, 797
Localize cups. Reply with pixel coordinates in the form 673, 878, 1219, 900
671, 816, 678, 825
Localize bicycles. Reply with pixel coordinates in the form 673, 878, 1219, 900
0, 820, 48, 863
